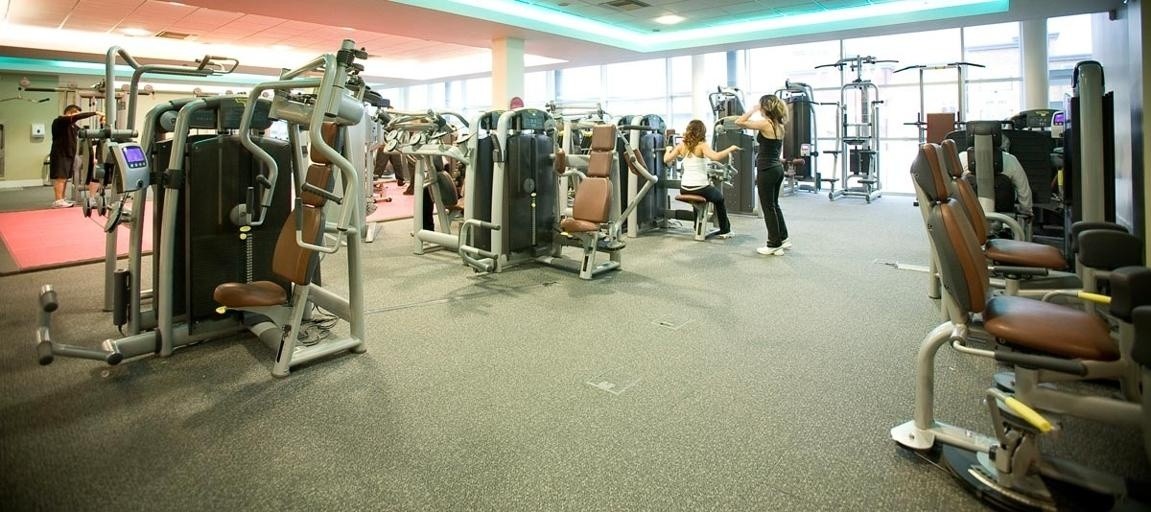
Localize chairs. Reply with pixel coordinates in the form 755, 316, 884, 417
213, 122, 339, 309
431, 154, 467, 213
562, 123, 617, 236
941, 141, 1066, 277
913, 140, 1121, 363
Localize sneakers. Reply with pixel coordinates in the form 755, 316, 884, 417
757, 238, 792, 256
89, 198, 98, 209
51, 199, 75, 209
719, 232, 735, 239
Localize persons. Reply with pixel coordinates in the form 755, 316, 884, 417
664, 120, 741, 239
952, 133, 1036, 216
50, 104, 106, 208
373, 99, 407, 186
424, 154, 458, 230
734, 95, 792, 255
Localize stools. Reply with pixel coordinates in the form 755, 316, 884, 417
675, 192, 712, 240
